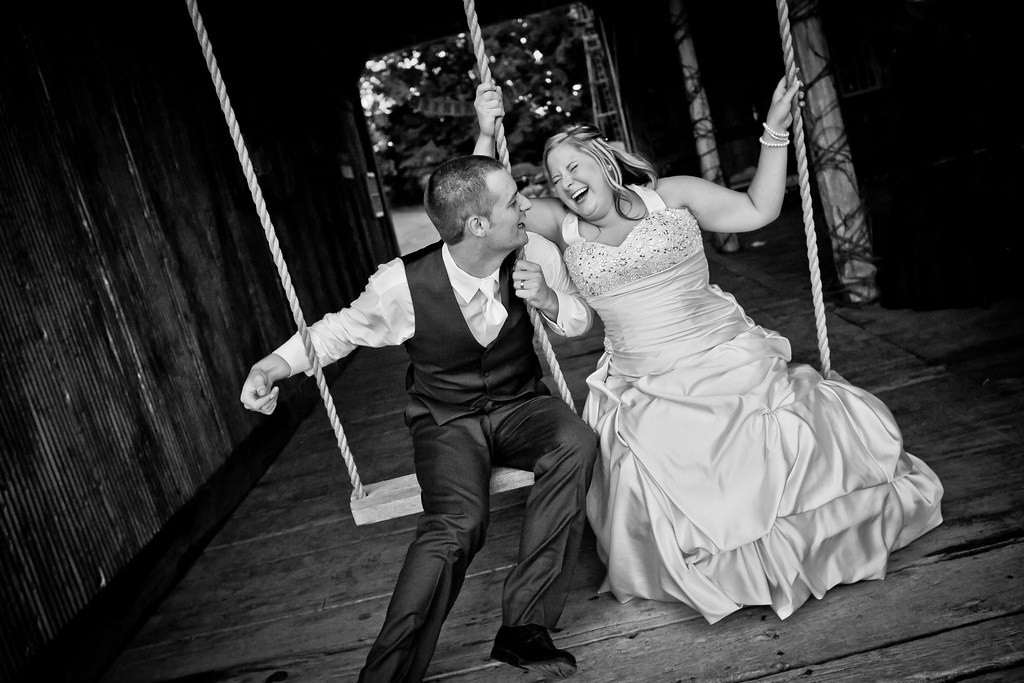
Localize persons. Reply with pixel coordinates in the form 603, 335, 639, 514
239, 151, 599, 682
472, 65, 948, 625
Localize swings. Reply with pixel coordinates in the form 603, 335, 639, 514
182, 0, 833, 529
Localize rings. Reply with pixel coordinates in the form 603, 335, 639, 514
520, 280, 526, 290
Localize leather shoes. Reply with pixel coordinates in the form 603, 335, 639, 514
489, 623, 577, 680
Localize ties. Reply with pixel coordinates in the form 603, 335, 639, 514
477, 279, 508, 346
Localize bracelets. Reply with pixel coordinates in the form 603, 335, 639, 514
758, 119, 791, 149
